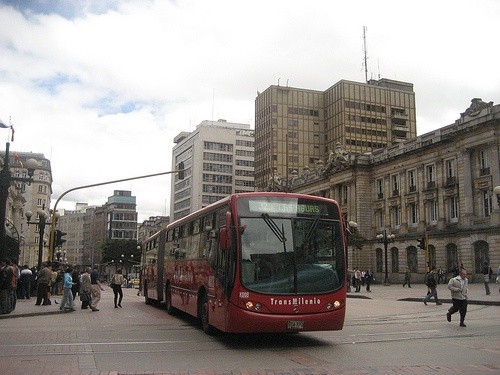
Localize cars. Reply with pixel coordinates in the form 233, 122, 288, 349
127, 278, 140, 289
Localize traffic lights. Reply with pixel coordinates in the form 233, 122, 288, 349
56, 229, 67, 245
417, 237, 426, 250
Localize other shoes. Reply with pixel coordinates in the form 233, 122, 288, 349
115, 306, 119, 308
82, 306, 89, 309
436, 302, 442, 305
355, 290, 359, 292
460, 323, 466, 327
60, 307, 62, 310
70, 309, 76, 311
368, 290, 371, 292
447, 313, 451, 323
92, 309, 99, 311
118, 304, 122, 308
424, 299, 427, 305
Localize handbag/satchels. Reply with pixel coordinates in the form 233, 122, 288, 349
80, 291, 92, 301
110, 275, 116, 288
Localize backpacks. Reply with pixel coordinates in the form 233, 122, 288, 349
425, 273, 432, 286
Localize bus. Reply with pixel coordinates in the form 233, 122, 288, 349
139, 191, 359, 337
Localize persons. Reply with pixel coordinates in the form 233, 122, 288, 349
361, 270, 366, 285
0, 258, 20, 314
21, 265, 33, 299
403, 266, 411, 288
355, 267, 362, 292
34, 265, 51, 305
113, 269, 124, 308
365, 269, 373, 292
56, 269, 65, 295
437, 266, 444, 284
481, 260, 493, 295
446, 269, 468, 327
51, 269, 58, 295
81, 267, 93, 308
89, 264, 104, 311
60, 268, 76, 310
72, 268, 81, 300
423, 267, 442, 306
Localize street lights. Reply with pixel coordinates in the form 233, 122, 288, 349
119, 254, 134, 279
0, 141, 39, 263
376, 229, 396, 286
25, 208, 61, 268
